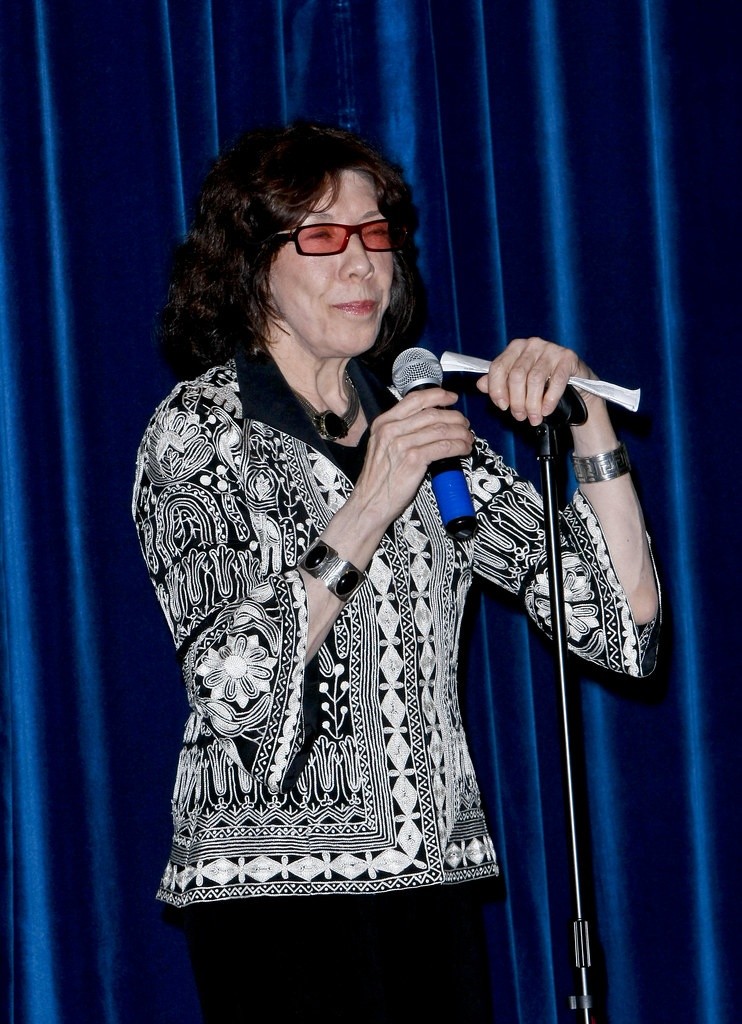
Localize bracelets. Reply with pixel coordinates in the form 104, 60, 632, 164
570, 441, 631, 483
295, 538, 367, 604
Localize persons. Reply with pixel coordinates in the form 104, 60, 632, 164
131, 120, 665, 1024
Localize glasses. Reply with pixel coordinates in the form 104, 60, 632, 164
274, 218, 409, 256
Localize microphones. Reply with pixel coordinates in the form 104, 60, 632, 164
392, 348, 478, 541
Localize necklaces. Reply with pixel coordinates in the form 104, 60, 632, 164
290, 370, 361, 441
